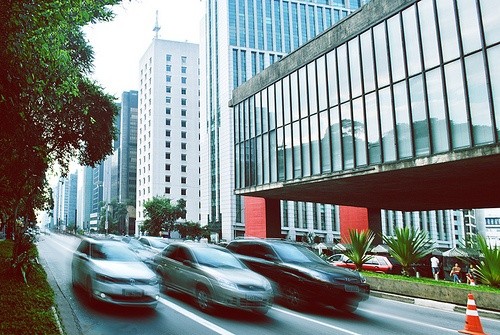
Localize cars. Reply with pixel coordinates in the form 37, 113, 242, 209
71, 238, 158, 307
326, 253, 350, 265
336, 254, 402, 277
144, 242, 274, 313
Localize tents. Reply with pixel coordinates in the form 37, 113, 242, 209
443, 248, 479, 281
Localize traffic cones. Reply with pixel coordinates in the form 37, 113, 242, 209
456, 294, 487, 335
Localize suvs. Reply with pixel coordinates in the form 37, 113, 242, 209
227, 237, 371, 312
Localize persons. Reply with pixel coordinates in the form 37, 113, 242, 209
450, 263, 461, 283
466, 265, 475, 284
431, 256, 439, 277
